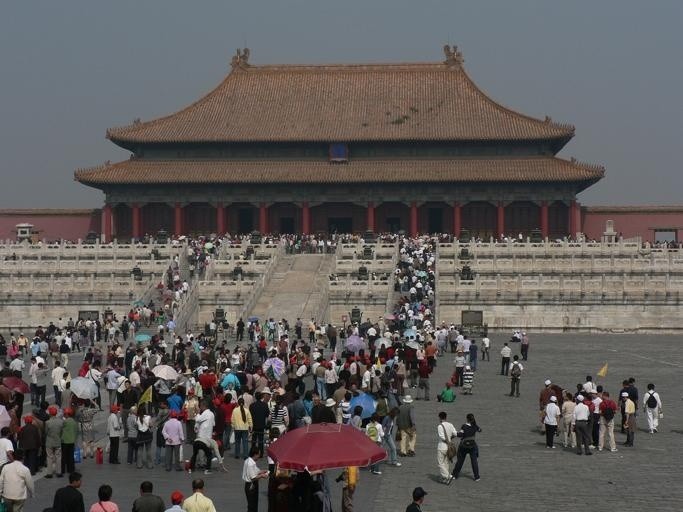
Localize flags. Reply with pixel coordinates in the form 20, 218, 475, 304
596, 361, 608, 377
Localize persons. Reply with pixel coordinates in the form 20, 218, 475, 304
182, 479, 216, 512
129, 481, 165, 512
405, 485, 427, 511
497, 231, 523, 244
89, 482, 120, 512
640, 237, 682, 249
0, 447, 35, 512
163, 489, 186, 512
239, 444, 268, 511
533, 376, 662, 458
480, 333, 492, 362
53, 473, 86, 512
510, 330, 529, 362
334, 464, 360, 511
450, 412, 484, 482
432, 412, 457, 485
496, 342, 510, 377
506, 355, 524, 399
266, 458, 332, 511
567, 234, 575, 243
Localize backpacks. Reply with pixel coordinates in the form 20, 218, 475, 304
603, 407, 615, 422
512, 364, 521, 377
647, 392, 658, 408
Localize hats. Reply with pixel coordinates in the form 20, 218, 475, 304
171, 491, 183, 504
413, 487, 427, 499
457, 331, 527, 370
0, 331, 441, 435
544, 379, 630, 404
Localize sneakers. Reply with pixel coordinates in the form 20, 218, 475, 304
110, 457, 215, 476
442, 473, 458, 485
372, 469, 383, 476
540, 428, 635, 455
473, 476, 481, 482
390, 461, 401, 467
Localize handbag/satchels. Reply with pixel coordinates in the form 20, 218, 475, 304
658, 411, 664, 419
447, 442, 457, 457
